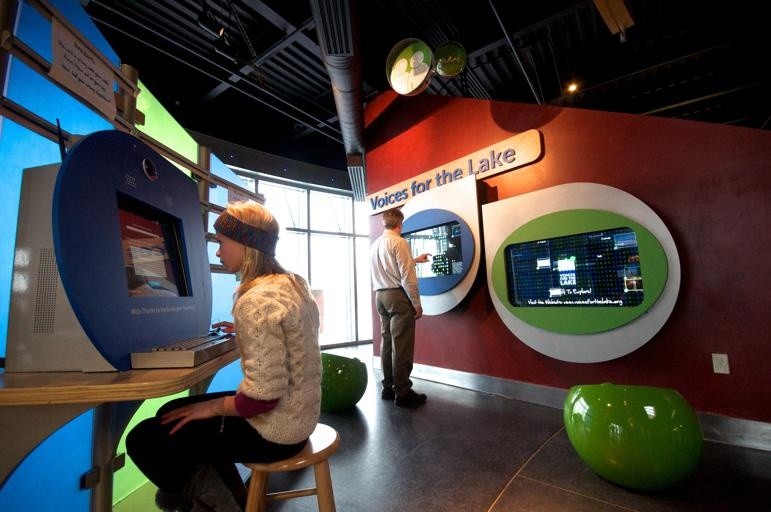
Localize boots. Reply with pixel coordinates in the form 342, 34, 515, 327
185, 460, 246, 512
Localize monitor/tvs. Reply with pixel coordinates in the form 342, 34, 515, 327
400, 222, 463, 278
506, 226, 644, 307
118, 206, 189, 298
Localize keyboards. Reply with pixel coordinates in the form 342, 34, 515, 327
130, 335, 238, 368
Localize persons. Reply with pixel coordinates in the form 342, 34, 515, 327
125, 199, 323, 511
369, 207, 432, 406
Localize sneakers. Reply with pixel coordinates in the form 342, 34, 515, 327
382, 388, 427, 407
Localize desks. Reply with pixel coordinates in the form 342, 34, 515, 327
2, 347, 243, 510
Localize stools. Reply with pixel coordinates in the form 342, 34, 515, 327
240, 421, 340, 511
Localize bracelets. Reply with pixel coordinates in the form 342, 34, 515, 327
217, 395, 228, 433
